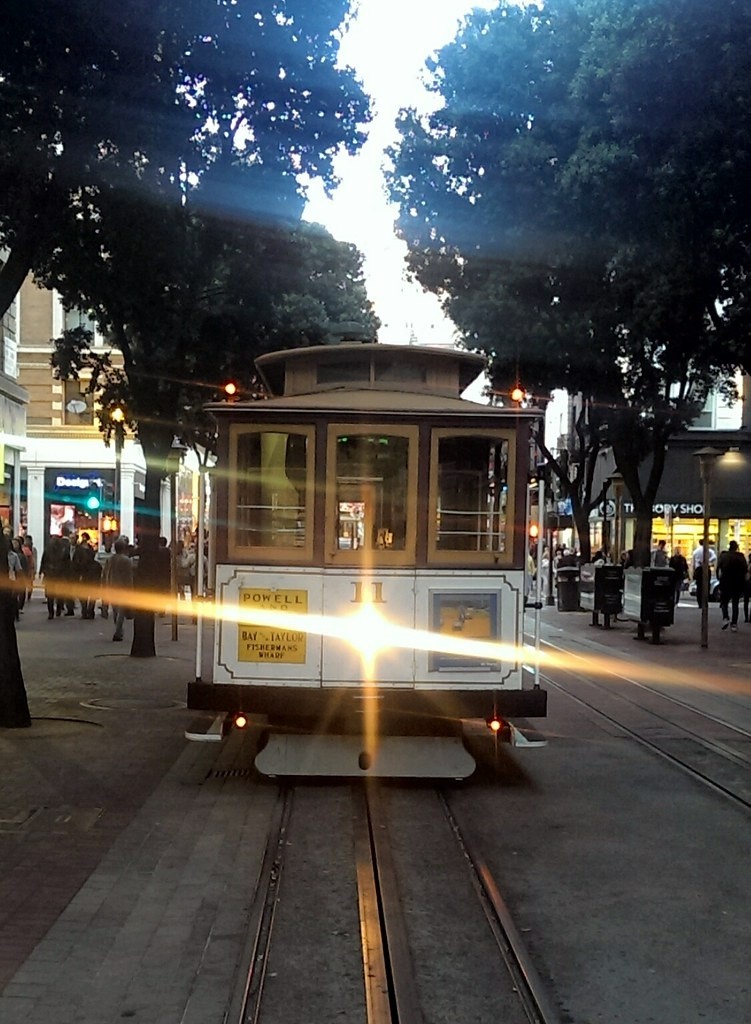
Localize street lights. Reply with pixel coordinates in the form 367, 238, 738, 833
691, 445, 726, 647
166, 445, 190, 644
605, 473, 626, 563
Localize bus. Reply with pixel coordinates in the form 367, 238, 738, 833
185, 342, 548, 781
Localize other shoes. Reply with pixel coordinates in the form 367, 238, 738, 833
19, 609, 24, 613
721, 620, 729, 630
62, 606, 64, 610
113, 637, 123, 641
730, 623, 737, 631
100, 607, 108, 619
49, 609, 54, 619
56, 609, 61, 616
65, 613, 74, 616
81, 610, 95, 619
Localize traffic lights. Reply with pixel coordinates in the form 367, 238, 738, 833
88, 482, 99, 513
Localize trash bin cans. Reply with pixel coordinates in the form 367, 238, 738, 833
593, 564, 625, 616
640, 567, 677, 627
555, 566, 582, 611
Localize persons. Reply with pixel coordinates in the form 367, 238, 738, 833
156, 528, 210, 617
38, 527, 139, 641
4, 526, 38, 621
524, 541, 581, 612
650, 540, 688, 606
716, 540, 751, 630
620, 549, 634, 590
591, 544, 613, 564
692, 539, 716, 609
